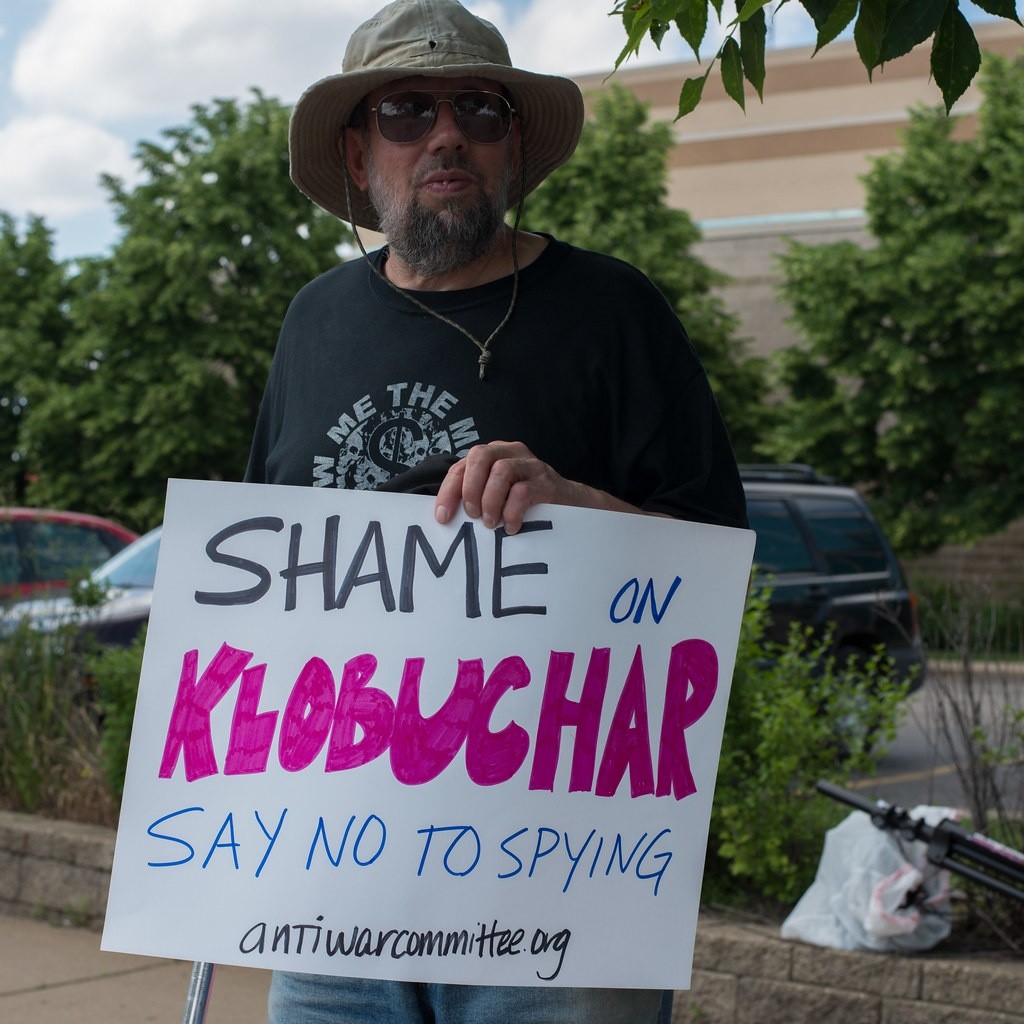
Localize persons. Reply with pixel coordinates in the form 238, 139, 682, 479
239, 1, 755, 1024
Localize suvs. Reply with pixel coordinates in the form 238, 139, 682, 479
737, 463, 927, 768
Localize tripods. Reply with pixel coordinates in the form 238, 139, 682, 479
814, 778, 1023, 903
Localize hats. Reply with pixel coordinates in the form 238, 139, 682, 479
288, 0, 583, 232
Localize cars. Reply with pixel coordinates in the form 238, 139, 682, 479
3, 522, 164, 634
0, 509, 142, 606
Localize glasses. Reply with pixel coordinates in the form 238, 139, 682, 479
351, 91, 520, 145
